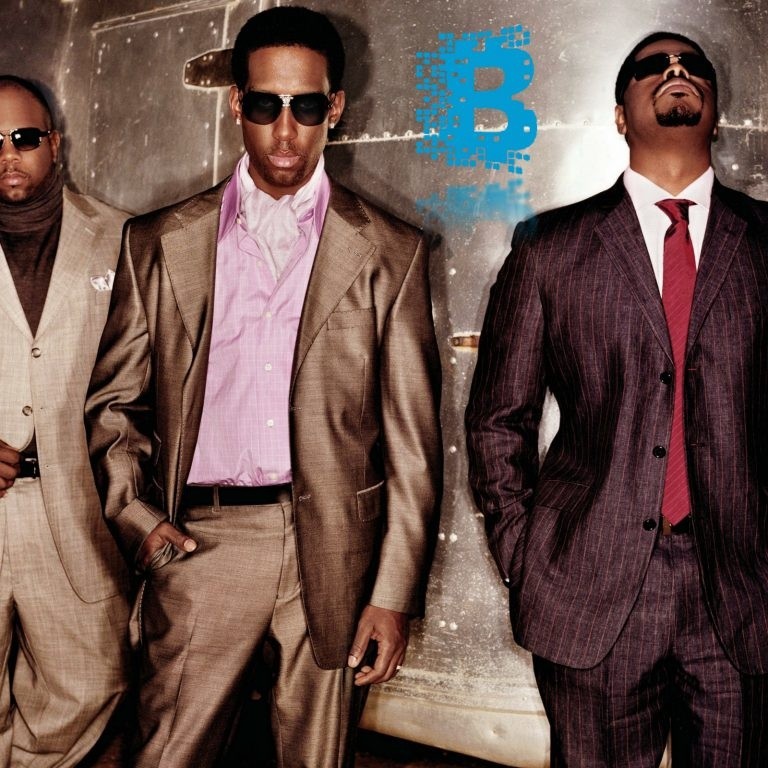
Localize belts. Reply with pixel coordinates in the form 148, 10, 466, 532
660, 513, 693, 537
17, 460, 40, 479
183, 483, 290, 506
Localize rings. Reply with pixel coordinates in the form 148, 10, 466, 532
396, 664, 401, 671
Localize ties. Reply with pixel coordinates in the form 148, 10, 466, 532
656, 199, 696, 526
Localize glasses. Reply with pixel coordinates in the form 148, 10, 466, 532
0, 128, 51, 151
242, 92, 331, 126
631, 52, 715, 81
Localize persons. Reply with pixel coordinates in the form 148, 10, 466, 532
0, 74, 142, 767
84, 4, 445, 767
463, 32, 767, 767
